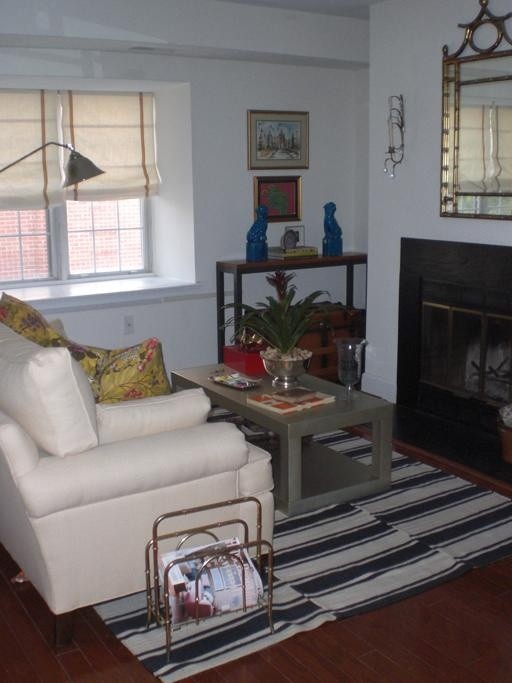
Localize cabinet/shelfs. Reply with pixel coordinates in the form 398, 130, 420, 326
215, 251, 370, 363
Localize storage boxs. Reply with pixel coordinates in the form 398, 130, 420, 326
221, 343, 267, 376
245, 300, 366, 386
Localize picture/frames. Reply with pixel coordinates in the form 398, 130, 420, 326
245, 106, 312, 170
252, 172, 304, 224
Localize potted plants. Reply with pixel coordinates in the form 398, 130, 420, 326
218, 267, 357, 389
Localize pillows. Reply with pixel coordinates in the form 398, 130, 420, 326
0, 289, 102, 374
76, 335, 172, 403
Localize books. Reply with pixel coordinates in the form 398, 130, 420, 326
267, 245, 318, 261
206, 405, 280, 444
246, 385, 336, 416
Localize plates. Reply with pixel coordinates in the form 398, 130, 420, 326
206, 370, 263, 390
283, 228, 298, 249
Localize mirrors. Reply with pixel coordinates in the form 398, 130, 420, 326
439, 0, 511, 222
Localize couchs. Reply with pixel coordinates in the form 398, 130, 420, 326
0, 303, 277, 656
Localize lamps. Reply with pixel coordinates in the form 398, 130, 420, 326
0, 139, 106, 188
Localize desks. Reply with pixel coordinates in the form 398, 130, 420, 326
169, 360, 396, 517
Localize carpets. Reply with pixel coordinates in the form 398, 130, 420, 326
90, 400, 511, 682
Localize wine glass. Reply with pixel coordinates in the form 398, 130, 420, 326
334, 337, 368, 401
